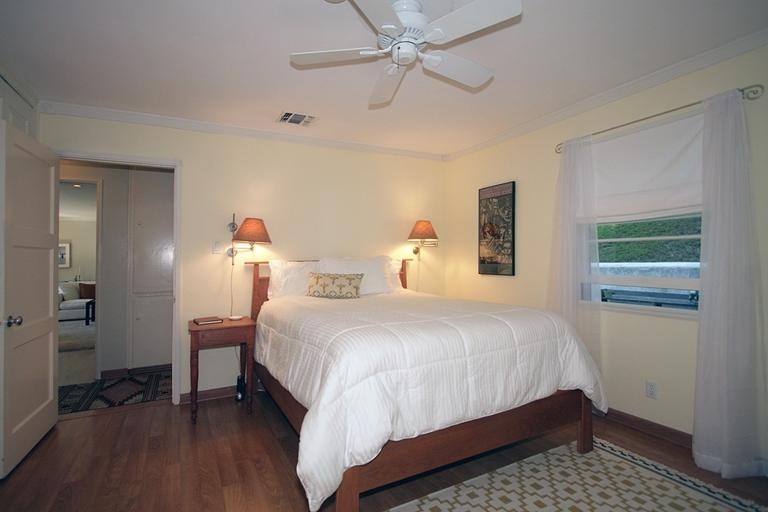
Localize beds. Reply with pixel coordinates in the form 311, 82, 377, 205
243, 258, 593, 512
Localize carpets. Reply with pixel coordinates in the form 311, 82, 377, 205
385, 434, 768, 512
57, 369, 172, 415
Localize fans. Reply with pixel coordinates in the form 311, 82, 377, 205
287, 0, 524, 106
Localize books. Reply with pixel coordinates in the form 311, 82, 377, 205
191, 316, 222, 326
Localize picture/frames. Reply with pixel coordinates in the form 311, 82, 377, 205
57, 242, 71, 269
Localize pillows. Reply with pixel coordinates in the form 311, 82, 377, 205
266, 255, 403, 300
79, 282, 96, 300
60, 282, 80, 301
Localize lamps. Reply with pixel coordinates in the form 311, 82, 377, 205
407, 219, 440, 254
227, 217, 272, 257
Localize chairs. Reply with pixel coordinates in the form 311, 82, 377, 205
57, 281, 96, 323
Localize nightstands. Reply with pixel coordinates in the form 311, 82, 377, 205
187, 316, 257, 425
